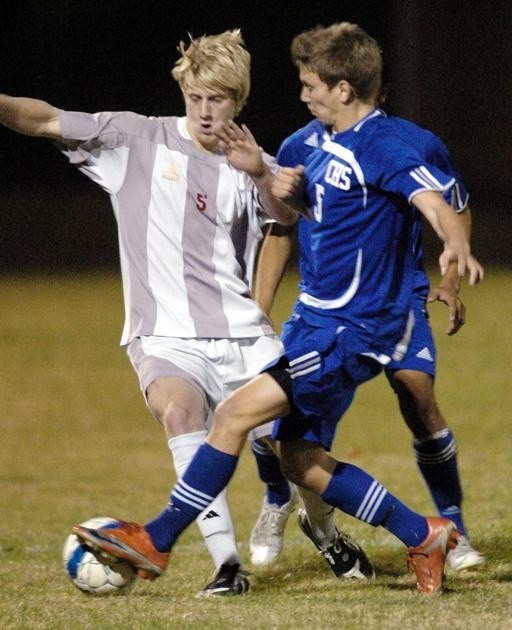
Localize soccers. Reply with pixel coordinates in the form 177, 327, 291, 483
63, 515, 137, 594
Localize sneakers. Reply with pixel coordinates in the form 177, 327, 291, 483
194, 562, 257, 599
406, 516, 456, 600
70, 519, 171, 582
446, 532, 488, 572
249, 485, 298, 567
297, 502, 379, 585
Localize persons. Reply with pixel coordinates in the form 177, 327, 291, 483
70, 20, 491, 599
0, 25, 377, 601
248, 66, 489, 576
